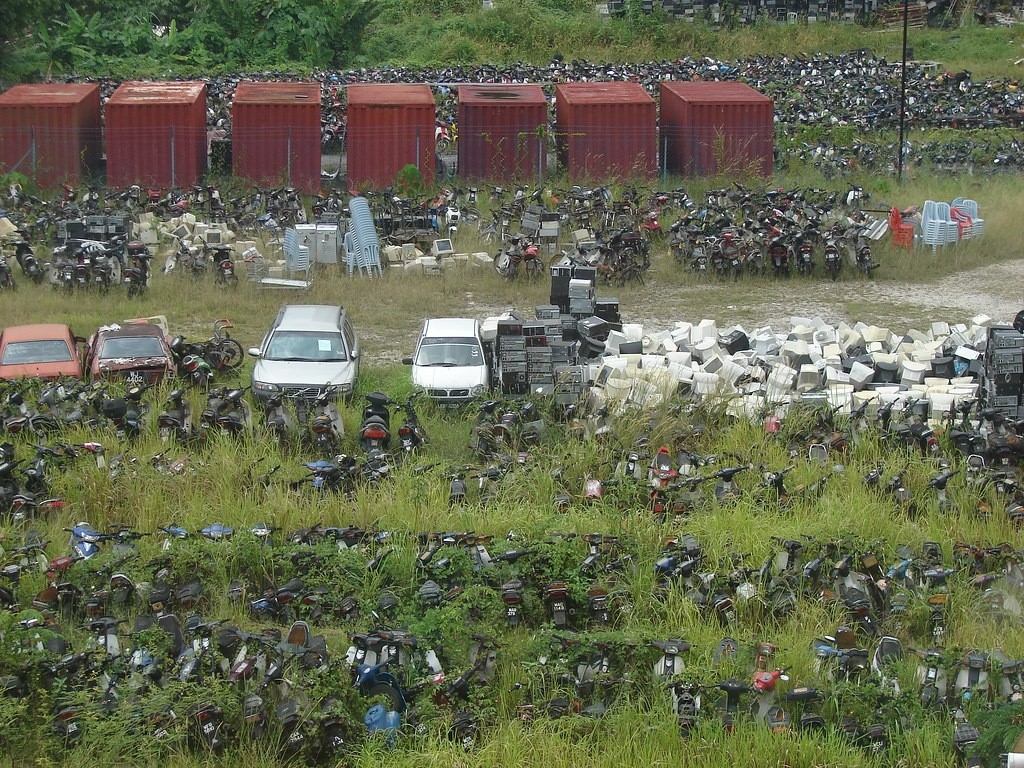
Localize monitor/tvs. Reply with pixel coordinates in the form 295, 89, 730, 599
57, 212, 287, 280
383, 239, 494, 276
581, 314, 1012, 434
0, 218, 18, 238
559, 228, 596, 254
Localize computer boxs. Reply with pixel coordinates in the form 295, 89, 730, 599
596, 0, 879, 28
978, 325, 1024, 422
481, 265, 623, 422
520, 204, 560, 262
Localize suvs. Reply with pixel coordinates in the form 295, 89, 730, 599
247, 303, 361, 406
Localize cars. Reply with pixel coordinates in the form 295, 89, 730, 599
402, 317, 494, 410
0, 322, 86, 383
83, 324, 177, 389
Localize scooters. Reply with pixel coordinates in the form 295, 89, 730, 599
0, 0, 1024, 768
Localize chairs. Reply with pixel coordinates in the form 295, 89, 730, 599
282, 226, 309, 278
343, 197, 383, 282
889, 196, 984, 256
